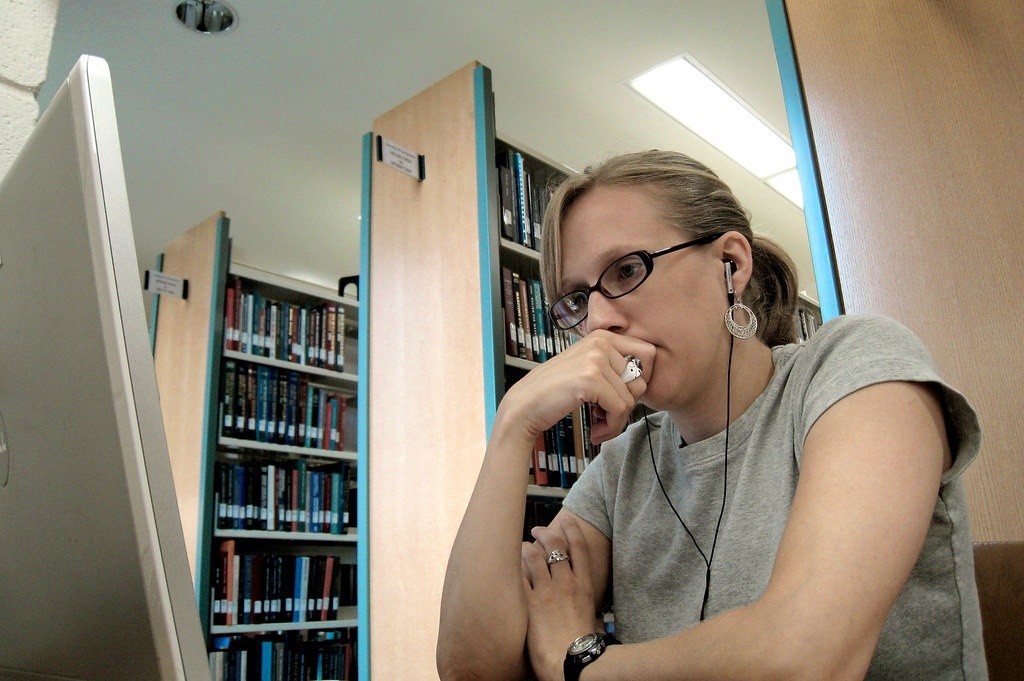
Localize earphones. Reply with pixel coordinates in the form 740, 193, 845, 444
721, 258, 737, 308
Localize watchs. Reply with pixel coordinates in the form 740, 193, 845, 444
563, 632, 624, 681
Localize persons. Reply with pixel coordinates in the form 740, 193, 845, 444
436, 149, 989, 681
496, 140, 620, 629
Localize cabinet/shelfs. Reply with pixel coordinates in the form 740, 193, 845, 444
149, 0, 1024, 681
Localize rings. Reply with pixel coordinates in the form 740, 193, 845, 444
547, 549, 571, 568
621, 359, 644, 384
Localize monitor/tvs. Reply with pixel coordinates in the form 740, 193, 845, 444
0, 54, 213, 681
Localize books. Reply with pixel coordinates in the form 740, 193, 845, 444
784, 305, 825, 344
208, 271, 359, 680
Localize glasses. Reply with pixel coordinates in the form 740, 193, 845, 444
548, 232, 724, 331
